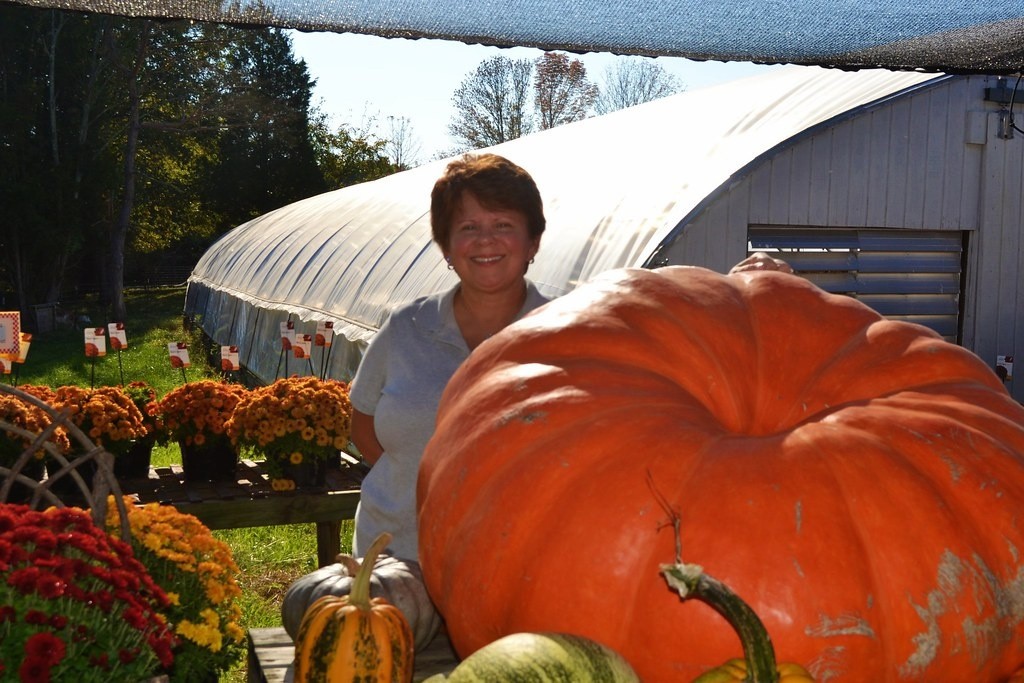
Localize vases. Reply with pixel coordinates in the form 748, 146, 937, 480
2, 450, 152, 504
177, 441, 238, 481
267, 457, 326, 488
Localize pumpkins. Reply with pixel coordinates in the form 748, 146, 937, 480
294, 531, 415, 683
282, 554, 443, 655
416, 265, 1024, 683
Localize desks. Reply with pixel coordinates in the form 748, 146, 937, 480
245, 616, 463, 683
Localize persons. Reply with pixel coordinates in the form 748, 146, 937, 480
345, 153, 569, 571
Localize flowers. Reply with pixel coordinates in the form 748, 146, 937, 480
0, 382, 159, 463
0, 494, 247, 683
223, 374, 355, 492
150, 379, 250, 455
